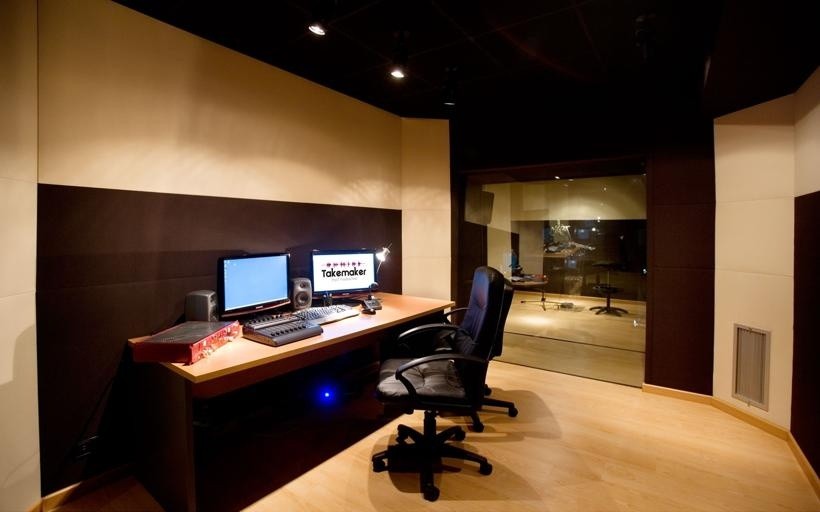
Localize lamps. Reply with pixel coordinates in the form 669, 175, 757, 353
370, 241, 395, 301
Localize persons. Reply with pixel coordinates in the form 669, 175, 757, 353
510, 245, 518, 277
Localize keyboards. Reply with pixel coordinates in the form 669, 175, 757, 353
292, 304, 360, 325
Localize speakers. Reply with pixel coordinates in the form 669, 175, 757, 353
185, 290, 219, 321
291, 278, 312, 309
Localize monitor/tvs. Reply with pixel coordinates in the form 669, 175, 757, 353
311, 249, 378, 296
216, 252, 291, 315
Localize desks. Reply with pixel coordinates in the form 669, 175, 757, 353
127, 289, 457, 512
512, 278, 549, 310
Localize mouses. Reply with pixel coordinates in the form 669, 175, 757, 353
362, 309, 375, 314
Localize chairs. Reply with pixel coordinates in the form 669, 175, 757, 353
589, 262, 627, 317
377, 267, 519, 502
